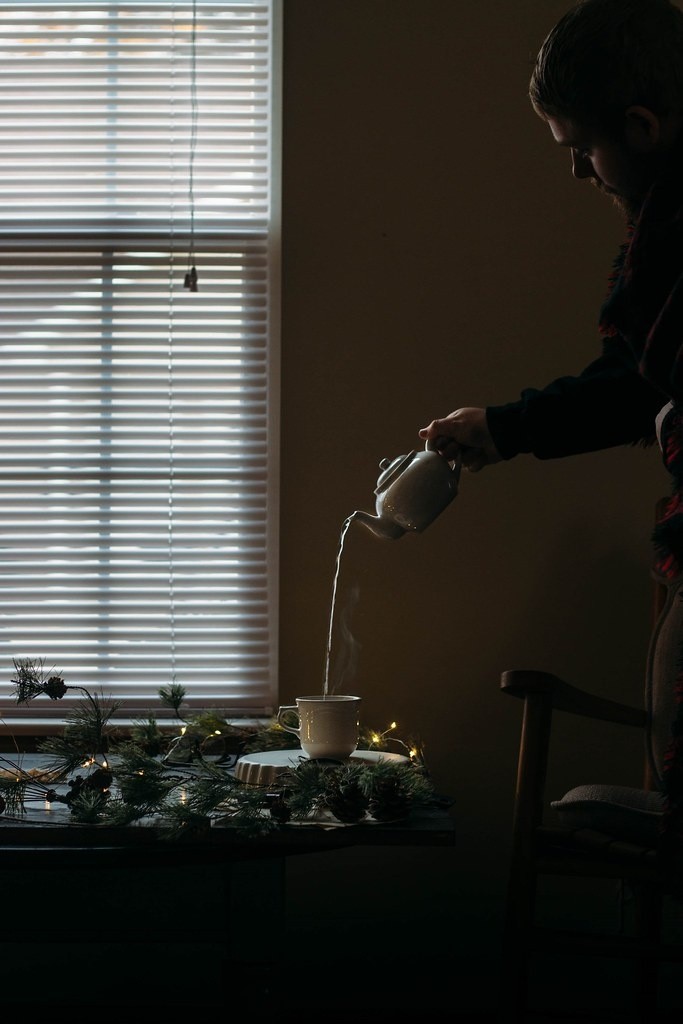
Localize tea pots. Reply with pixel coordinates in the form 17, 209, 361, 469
346, 429, 462, 540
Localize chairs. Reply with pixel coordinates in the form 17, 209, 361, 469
502, 500, 683, 1024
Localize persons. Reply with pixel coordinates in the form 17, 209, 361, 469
420, 0, 683, 900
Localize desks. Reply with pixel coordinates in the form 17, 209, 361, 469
0, 755, 463, 1024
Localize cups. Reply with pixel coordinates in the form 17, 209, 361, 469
278, 696, 362, 758
